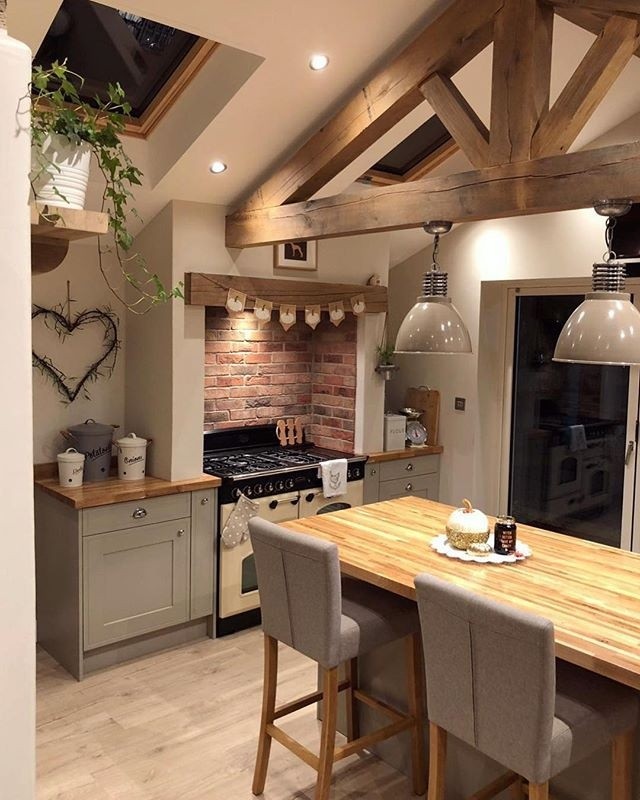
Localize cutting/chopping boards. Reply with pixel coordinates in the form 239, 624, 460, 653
404, 385, 440, 446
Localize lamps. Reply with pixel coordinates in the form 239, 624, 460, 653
393, 220, 473, 358
551, 199, 640, 366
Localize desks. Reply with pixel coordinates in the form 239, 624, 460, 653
279, 495, 640, 800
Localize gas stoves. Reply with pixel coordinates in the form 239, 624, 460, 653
203, 423, 368, 503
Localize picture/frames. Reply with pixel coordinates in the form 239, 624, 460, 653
273, 238, 318, 272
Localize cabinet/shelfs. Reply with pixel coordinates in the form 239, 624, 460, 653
379, 454, 440, 502
34, 487, 218, 682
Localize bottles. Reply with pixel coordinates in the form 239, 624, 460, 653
494, 515, 516, 556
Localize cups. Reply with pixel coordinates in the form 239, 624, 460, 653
57, 448, 84, 487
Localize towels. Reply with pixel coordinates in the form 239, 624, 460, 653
317, 458, 348, 498
567, 424, 587, 452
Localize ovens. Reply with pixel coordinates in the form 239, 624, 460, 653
217, 479, 364, 637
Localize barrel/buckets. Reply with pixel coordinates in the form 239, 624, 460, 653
60, 418, 120, 481
113, 433, 152, 480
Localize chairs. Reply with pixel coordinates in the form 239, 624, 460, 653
411, 570, 640, 800
247, 517, 426, 800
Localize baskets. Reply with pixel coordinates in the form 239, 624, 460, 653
445, 526, 490, 550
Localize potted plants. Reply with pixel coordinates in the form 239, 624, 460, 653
375, 342, 395, 381
27, 58, 188, 315
